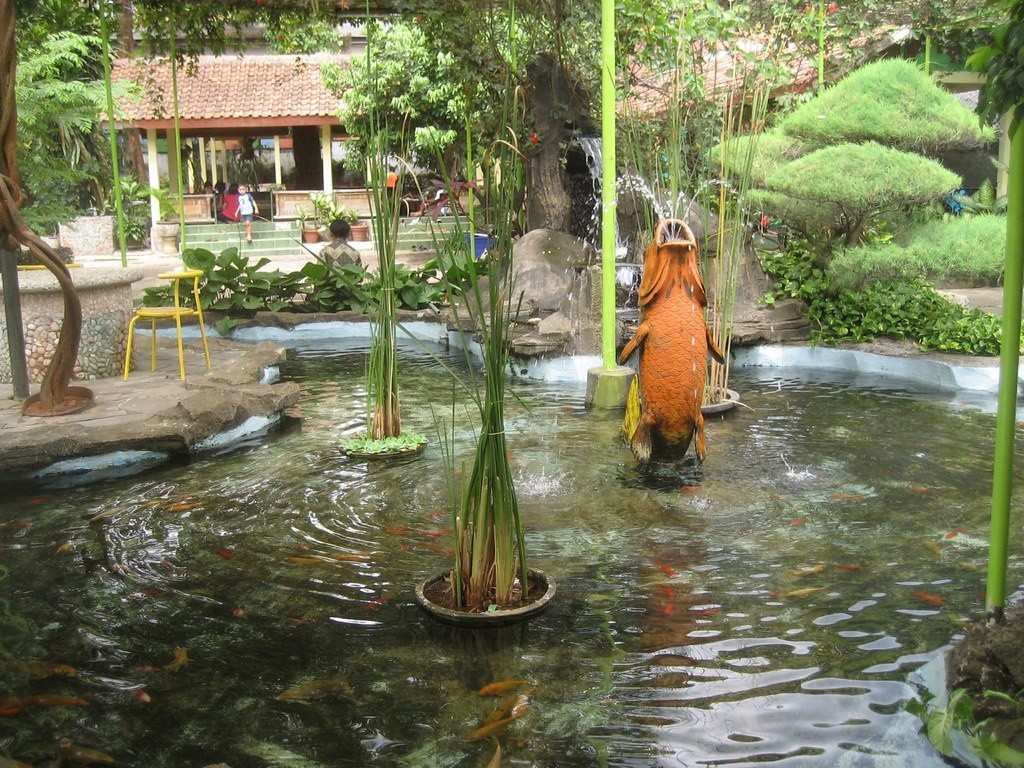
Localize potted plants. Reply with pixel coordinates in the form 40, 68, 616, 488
344, 209, 367, 241
294, 193, 317, 243
151, 186, 181, 253
100, 174, 148, 249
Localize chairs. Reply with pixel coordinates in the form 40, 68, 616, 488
123, 270, 212, 384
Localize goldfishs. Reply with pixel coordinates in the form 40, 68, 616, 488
778, 483, 972, 555
464, 675, 554, 768
617, 219, 725, 463
2, 486, 342, 768
343, 449, 534, 609
679, 483, 703, 494
958, 559, 987, 603
656, 555, 952, 638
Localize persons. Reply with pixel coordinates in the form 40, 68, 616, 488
209, 181, 227, 221
386, 167, 398, 214
217, 183, 241, 223
203, 181, 212, 194
233, 183, 259, 241
